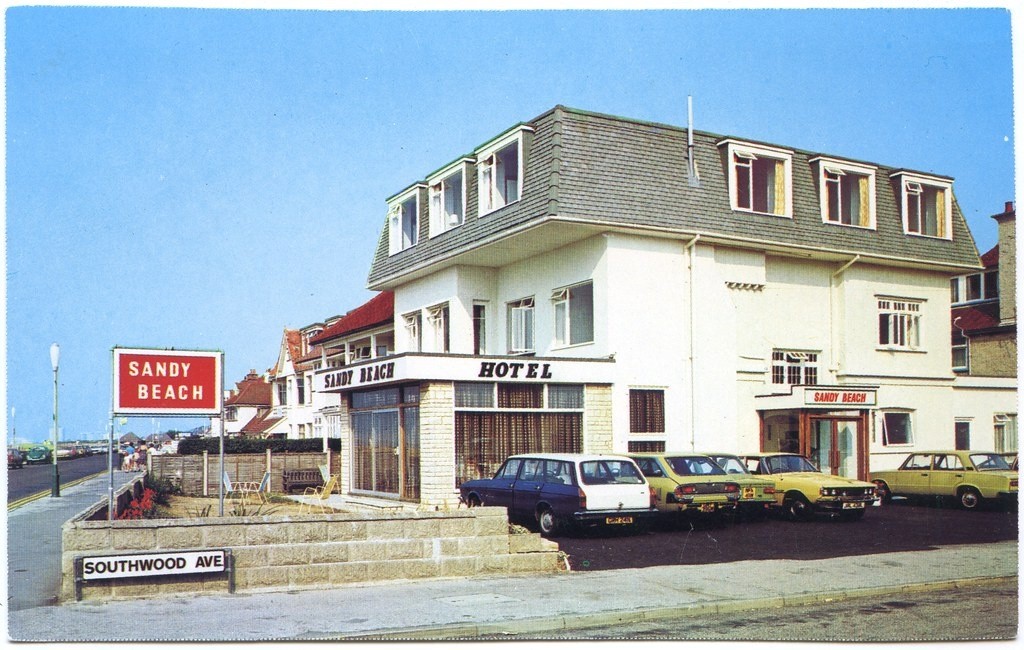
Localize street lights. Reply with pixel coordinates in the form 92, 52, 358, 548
11, 406, 16, 449
50, 342, 61, 499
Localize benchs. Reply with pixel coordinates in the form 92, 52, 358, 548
282, 468, 322, 494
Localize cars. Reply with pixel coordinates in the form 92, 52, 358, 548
75, 445, 129, 458
7, 448, 23, 469
595, 451, 1018, 525
51, 444, 76, 460
458, 453, 659, 538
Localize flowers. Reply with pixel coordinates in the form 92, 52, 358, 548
106, 488, 155, 520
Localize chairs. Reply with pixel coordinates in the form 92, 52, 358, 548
224, 471, 270, 506
298, 464, 342, 514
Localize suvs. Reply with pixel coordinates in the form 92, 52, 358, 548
26, 445, 52, 465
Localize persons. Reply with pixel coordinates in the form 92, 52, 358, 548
120, 437, 168, 473
